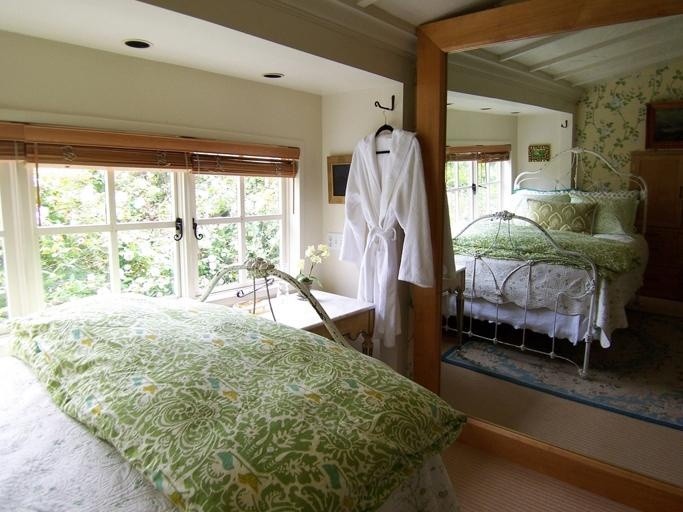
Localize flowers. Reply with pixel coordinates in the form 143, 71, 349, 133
294, 243, 329, 289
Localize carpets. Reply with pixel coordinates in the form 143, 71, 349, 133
440, 311, 680, 431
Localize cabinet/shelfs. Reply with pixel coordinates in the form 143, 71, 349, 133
628, 151, 682, 300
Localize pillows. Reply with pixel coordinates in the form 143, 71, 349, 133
508, 189, 640, 238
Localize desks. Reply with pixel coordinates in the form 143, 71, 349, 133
441, 264, 466, 350
231, 286, 375, 356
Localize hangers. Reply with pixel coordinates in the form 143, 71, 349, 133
369, 107, 397, 156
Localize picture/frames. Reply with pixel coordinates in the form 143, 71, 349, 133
645, 101, 682, 148
326, 156, 355, 204
528, 145, 550, 163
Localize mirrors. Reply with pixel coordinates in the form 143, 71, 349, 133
402, 1, 683, 508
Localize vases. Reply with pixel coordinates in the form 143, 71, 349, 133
298, 279, 312, 297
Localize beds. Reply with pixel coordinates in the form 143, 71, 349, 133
0, 261, 446, 511
439, 150, 648, 379
